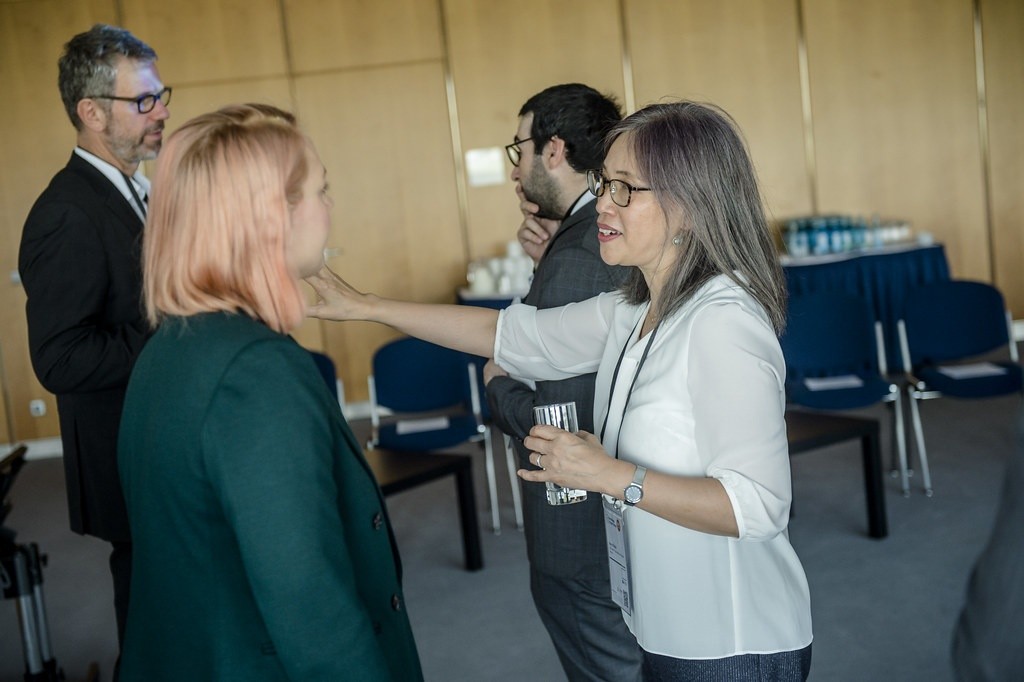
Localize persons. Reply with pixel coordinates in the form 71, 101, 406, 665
304, 103, 814, 682
18, 24, 173, 682
115, 104, 422, 682
480, 82, 643, 682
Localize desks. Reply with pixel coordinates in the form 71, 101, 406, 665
365, 448, 484, 573
783, 409, 890, 540
456, 241, 954, 409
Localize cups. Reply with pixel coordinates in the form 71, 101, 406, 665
534, 401, 587, 506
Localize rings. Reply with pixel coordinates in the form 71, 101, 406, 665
537, 455, 541, 467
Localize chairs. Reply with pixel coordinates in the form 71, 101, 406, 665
779, 294, 912, 500
306, 348, 348, 422
895, 277, 1024, 499
460, 298, 529, 531
366, 336, 502, 535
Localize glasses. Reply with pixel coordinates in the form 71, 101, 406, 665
505, 134, 558, 167
586, 166, 679, 208
87, 84, 172, 114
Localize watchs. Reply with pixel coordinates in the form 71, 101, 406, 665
624, 465, 647, 507
